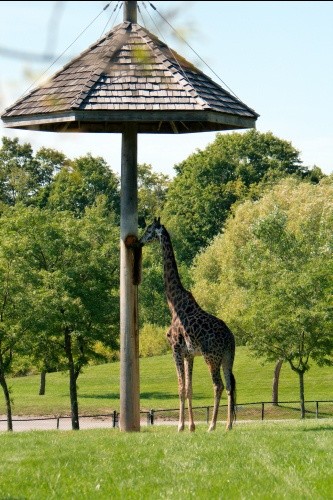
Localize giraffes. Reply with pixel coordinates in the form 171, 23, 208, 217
139, 216, 238, 434
